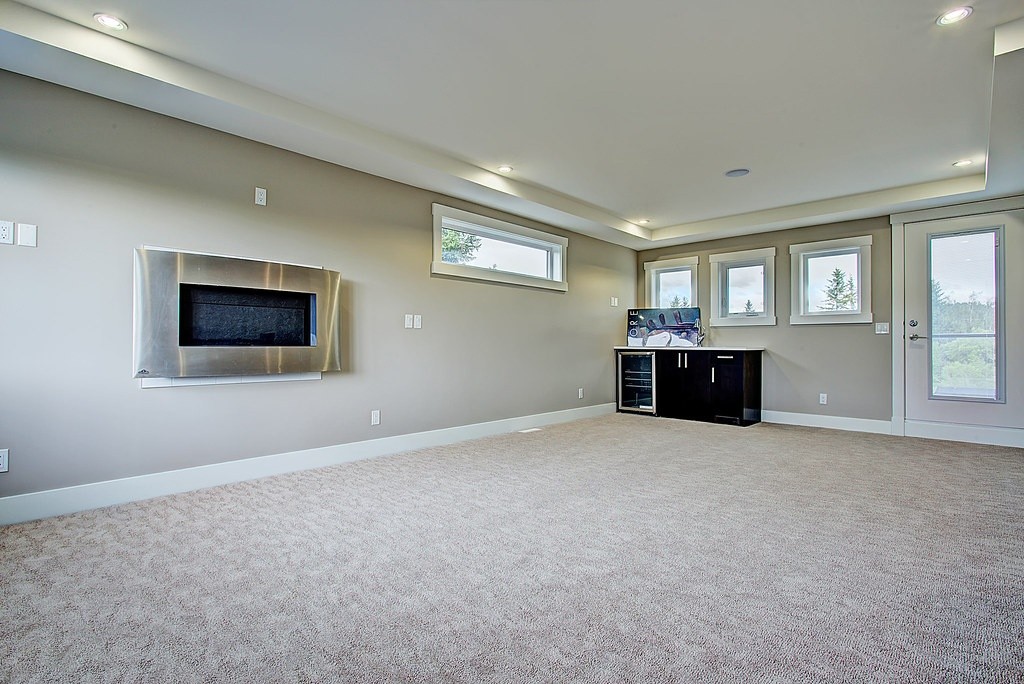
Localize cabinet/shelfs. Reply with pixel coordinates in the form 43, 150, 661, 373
613, 344, 765, 427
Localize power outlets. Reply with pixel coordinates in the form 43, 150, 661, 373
614, 298, 618, 307
0, 449, 9, 473
404, 314, 413, 328
579, 388, 583, 399
610, 296, 614, 306
0, 220, 15, 245
413, 314, 422, 328
254, 186, 267, 206
371, 410, 381, 425
819, 393, 827, 404
